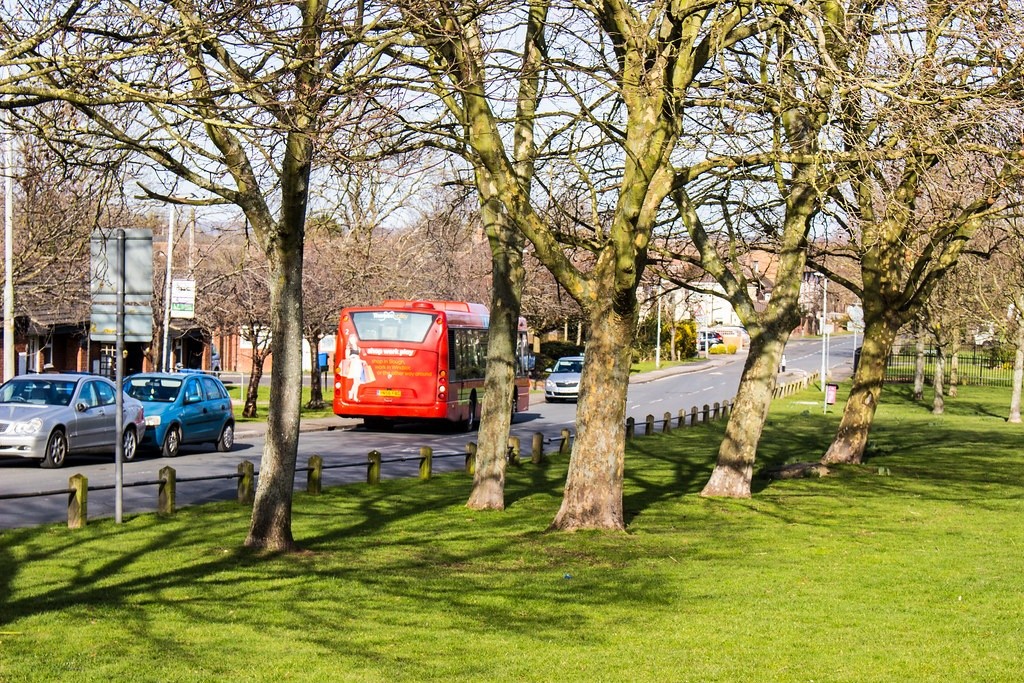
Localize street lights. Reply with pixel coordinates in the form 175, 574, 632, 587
814, 272, 827, 392
656, 280, 671, 369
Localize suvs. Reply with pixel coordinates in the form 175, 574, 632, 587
696, 331, 725, 350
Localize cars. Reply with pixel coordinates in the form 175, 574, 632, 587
544, 356, 585, 402
175, 345, 221, 378
974, 331, 994, 351
120, 372, 235, 456
0, 373, 146, 469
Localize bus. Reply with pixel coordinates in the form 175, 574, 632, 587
333, 299, 531, 428
